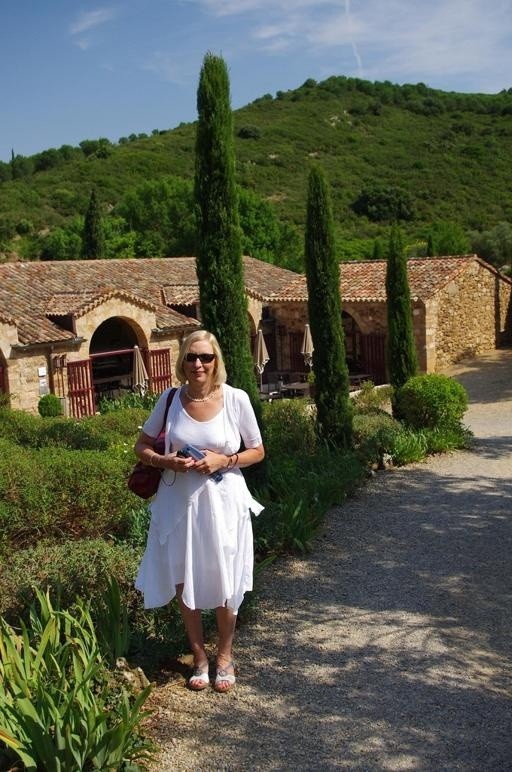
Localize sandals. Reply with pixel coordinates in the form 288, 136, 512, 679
214, 660, 236, 692
188, 661, 209, 690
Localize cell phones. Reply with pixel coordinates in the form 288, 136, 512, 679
176, 450, 187, 458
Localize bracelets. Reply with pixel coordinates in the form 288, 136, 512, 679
234, 453, 239, 465
227, 457, 233, 467
150, 453, 157, 466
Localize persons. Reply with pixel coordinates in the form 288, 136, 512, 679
134, 330, 266, 692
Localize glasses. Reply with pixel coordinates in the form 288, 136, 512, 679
184, 353, 215, 363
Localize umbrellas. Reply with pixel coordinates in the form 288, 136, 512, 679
301, 324, 315, 372
132, 345, 149, 397
252, 329, 270, 393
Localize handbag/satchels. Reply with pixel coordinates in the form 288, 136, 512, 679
127, 431, 165, 499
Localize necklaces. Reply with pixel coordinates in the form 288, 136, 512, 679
185, 385, 217, 401
